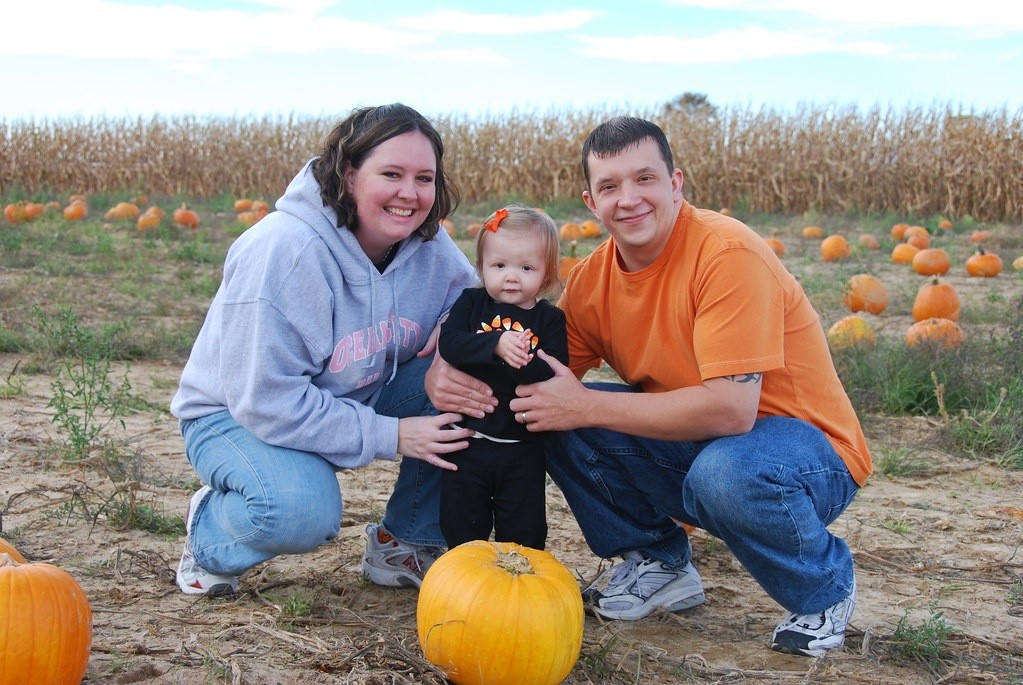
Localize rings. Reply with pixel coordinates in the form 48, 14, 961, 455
429, 455, 436, 464
522, 411, 527, 423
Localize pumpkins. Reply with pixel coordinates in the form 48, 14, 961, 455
0, 537, 93, 685
4, 195, 271, 232
719, 207, 1023, 348
439, 219, 480, 239
556, 220, 601, 281
417, 540, 585, 685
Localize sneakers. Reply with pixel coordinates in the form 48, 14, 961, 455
362, 523, 446, 590
770, 560, 856, 657
582, 550, 705, 620
175, 484, 237, 596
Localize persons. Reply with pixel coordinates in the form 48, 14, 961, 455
424, 117, 872, 657
170, 103, 489, 596
439, 205, 568, 551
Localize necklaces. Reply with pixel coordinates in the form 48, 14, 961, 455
382, 243, 395, 262
